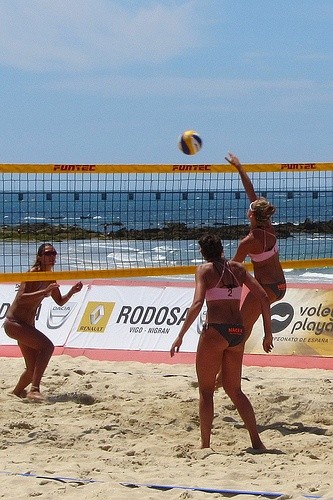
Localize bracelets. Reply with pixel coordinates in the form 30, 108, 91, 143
66, 294, 70, 299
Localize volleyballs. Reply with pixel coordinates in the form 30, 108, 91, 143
179, 131, 202, 154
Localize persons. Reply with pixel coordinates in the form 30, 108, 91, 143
170, 231, 275, 451
212, 151, 286, 392
2, 242, 83, 400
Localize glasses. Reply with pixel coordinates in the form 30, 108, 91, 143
39, 251, 57, 256
